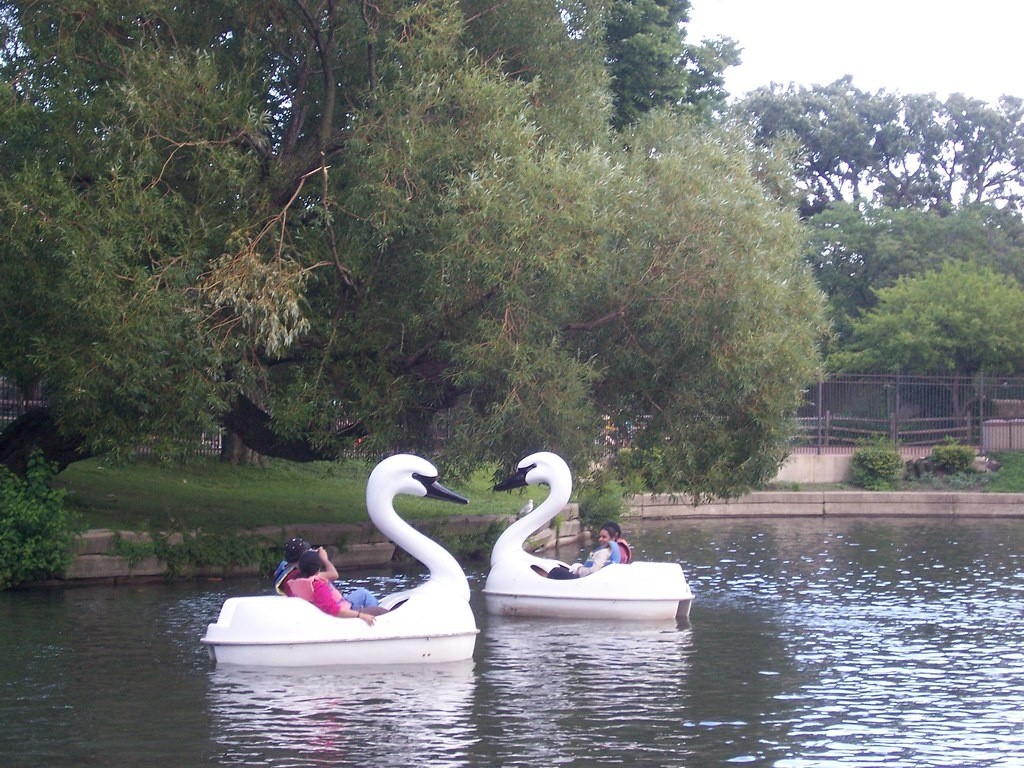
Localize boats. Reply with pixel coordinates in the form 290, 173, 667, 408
478, 452, 696, 619
198, 452, 482, 667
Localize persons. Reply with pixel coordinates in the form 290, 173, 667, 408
546, 522, 632, 579
274, 538, 390, 626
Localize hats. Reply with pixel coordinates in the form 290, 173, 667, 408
285, 538, 311, 560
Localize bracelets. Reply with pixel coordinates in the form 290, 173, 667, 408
357, 610, 361, 617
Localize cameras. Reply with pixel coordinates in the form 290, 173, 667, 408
308, 548, 319, 553
